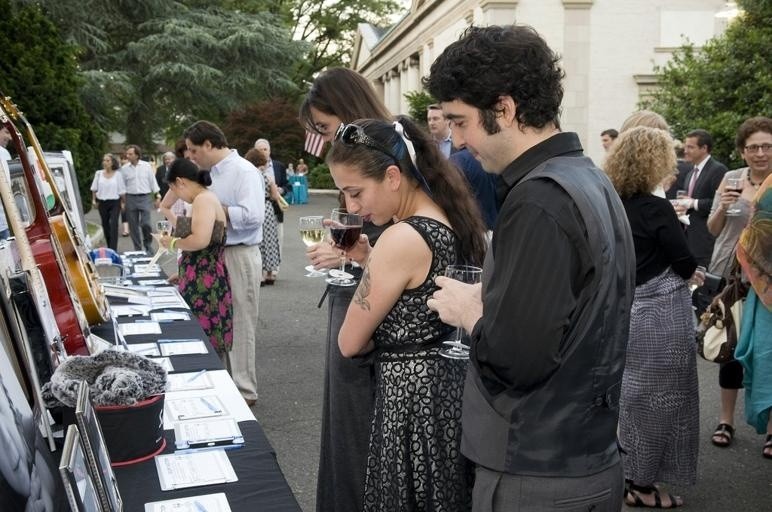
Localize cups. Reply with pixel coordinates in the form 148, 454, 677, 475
676, 190, 687, 199
156, 222, 173, 237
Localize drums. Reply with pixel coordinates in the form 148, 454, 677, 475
87, 248, 123, 281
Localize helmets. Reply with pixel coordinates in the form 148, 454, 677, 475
88, 247, 125, 278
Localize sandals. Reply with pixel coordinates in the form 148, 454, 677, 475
258, 272, 279, 288
712, 423, 736, 449
762, 434, 772, 458
623, 482, 684, 508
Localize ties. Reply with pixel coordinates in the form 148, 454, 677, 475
688, 168, 699, 199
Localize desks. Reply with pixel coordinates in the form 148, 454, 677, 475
45, 247, 308, 509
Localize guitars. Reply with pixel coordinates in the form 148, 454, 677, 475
0, 161, 72, 414
0, 95, 110, 357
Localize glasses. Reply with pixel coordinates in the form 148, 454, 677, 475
334, 122, 403, 174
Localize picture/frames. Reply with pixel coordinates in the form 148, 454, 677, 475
59, 422, 107, 512
0, 146, 89, 244
74, 380, 127, 511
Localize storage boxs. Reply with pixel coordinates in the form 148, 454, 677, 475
60, 393, 165, 463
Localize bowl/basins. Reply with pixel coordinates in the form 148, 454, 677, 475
73, 394, 166, 467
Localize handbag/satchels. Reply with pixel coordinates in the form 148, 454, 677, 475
698, 305, 731, 361
272, 199, 285, 223
692, 272, 728, 314
172, 214, 226, 246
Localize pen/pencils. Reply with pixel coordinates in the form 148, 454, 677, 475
188, 369, 206, 382
200, 398, 216, 411
157, 339, 200, 343
135, 320, 174, 323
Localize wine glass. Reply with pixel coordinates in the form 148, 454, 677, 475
688, 265, 707, 310
326, 208, 364, 287
300, 216, 327, 278
724, 179, 744, 217
439, 265, 483, 360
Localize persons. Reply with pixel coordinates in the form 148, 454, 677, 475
673, 129, 729, 271
705, 115, 771, 457
426, 104, 456, 161
183, 119, 266, 408
0, 119, 13, 237
425, 21, 637, 512
303, 68, 395, 381
620, 110, 669, 202
449, 149, 499, 230
601, 126, 706, 510
601, 129, 618, 150
158, 159, 234, 370
159, 141, 199, 259
732, 172, 772, 435
664, 139, 693, 201
89, 145, 311, 287
326, 117, 489, 512
